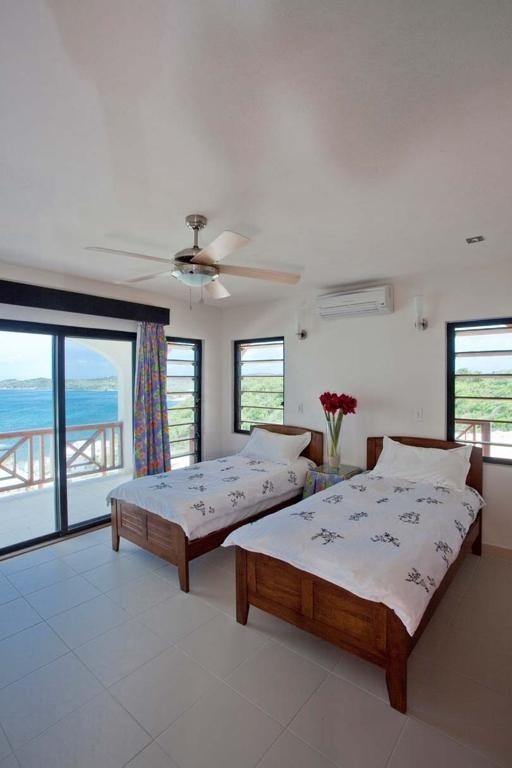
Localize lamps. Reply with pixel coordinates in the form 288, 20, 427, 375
292, 308, 307, 342
171, 266, 219, 312
413, 295, 430, 332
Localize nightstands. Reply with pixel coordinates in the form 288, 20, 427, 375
303, 463, 361, 500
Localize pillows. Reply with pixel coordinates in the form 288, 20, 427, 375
368, 435, 474, 493
238, 427, 312, 466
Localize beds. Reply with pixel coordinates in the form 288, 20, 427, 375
221, 437, 484, 712
109, 424, 324, 592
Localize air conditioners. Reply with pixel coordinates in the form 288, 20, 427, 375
317, 282, 392, 320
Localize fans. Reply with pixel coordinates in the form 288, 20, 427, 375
86, 214, 305, 301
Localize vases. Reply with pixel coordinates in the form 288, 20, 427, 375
327, 446, 340, 474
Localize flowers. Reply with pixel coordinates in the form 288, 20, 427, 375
318, 391, 357, 456
